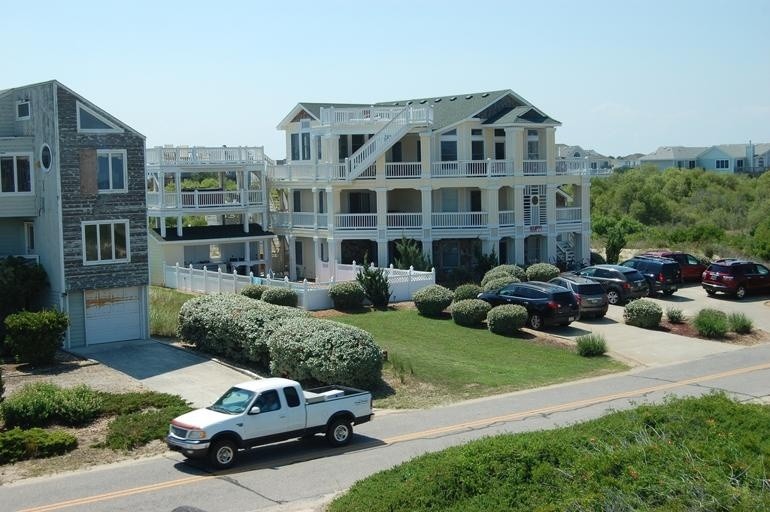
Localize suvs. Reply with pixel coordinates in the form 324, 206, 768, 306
618, 256, 683, 298
702, 258, 770, 300
544, 275, 608, 320
476, 280, 579, 330
640, 250, 709, 287
557, 262, 650, 306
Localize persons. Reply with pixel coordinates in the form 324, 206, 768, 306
262, 394, 279, 411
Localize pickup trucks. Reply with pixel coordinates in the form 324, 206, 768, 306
165, 375, 375, 470
